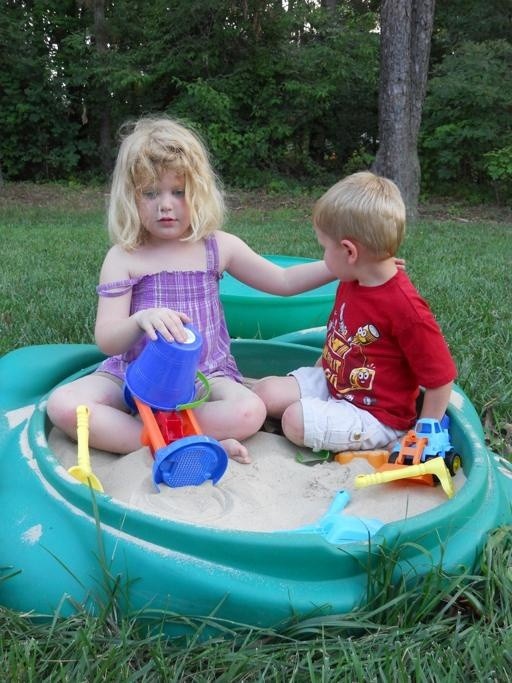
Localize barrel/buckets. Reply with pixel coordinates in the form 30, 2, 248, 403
119, 322, 213, 411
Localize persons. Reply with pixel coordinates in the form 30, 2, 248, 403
251, 170, 457, 455
47, 116, 406, 466
